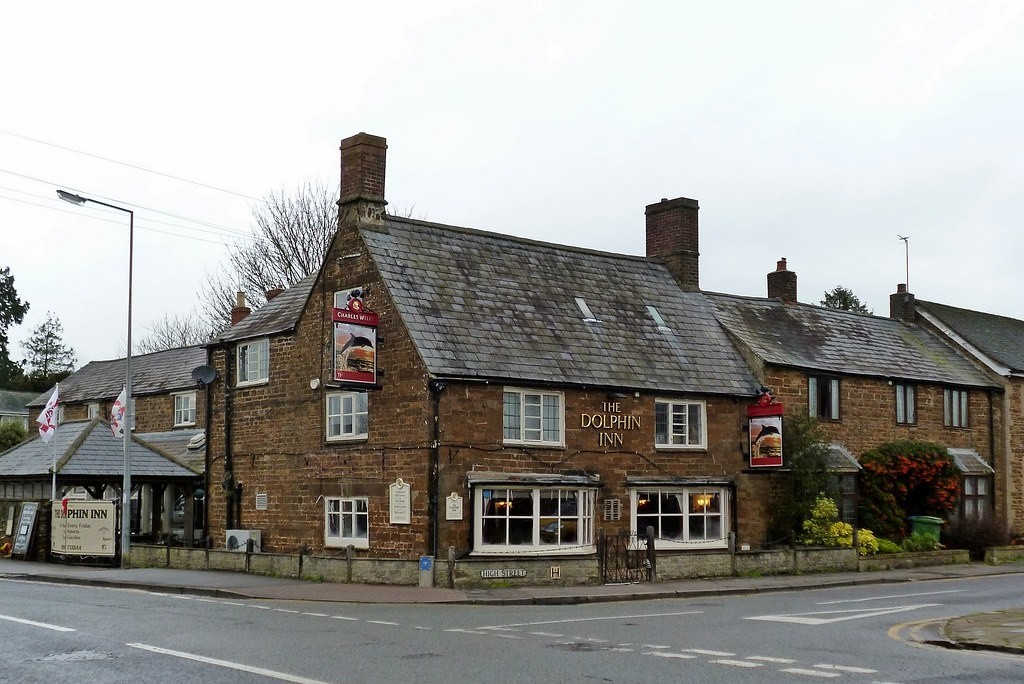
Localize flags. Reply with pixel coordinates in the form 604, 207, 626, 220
36, 386, 59, 444
110, 389, 126, 438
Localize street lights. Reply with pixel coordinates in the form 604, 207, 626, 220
56, 189, 134, 572
